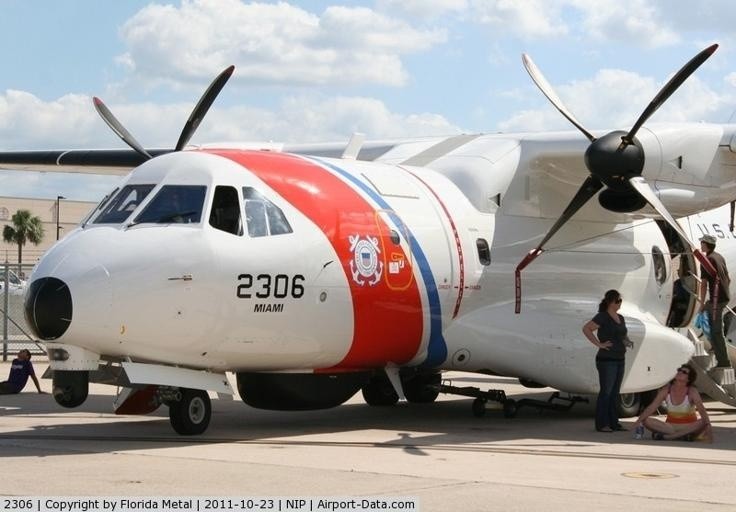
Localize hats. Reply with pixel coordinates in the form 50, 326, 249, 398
699, 234, 716, 245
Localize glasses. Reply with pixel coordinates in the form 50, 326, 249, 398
615, 299, 622, 303
677, 368, 686, 374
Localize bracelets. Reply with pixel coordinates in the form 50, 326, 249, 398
705, 421, 711, 424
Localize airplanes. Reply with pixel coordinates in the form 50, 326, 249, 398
0, 42, 736, 436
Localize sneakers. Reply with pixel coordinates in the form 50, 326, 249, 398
654, 433, 664, 440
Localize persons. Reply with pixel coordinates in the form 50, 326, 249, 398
583, 289, 634, 432
630, 364, 714, 443
0, 348, 47, 395
699, 233, 733, 368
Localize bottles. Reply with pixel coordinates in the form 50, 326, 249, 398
637, 420, 647, 441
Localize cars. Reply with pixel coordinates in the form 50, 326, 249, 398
0, 267, 27, 295
